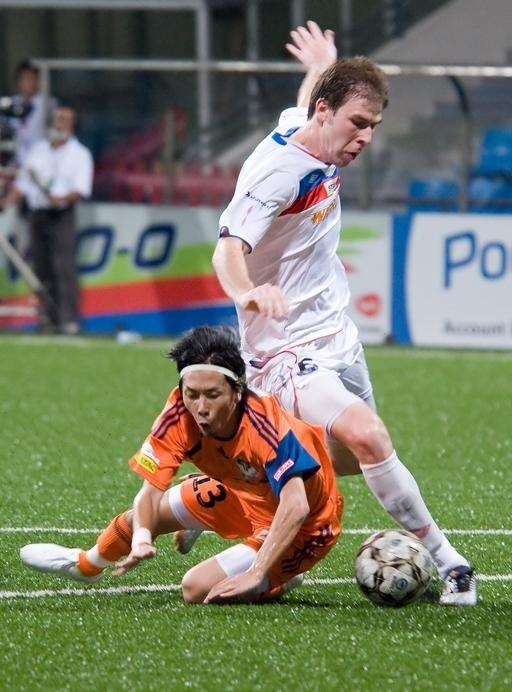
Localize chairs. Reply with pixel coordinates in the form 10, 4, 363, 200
408, 128, 511, 213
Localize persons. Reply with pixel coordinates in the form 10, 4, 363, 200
207, 19, 478, 610
1, 57, 58, 162
0, 106, 95, 334
20, 324, 344, 606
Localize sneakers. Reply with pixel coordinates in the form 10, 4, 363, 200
175, 473, 206, 553
40, 320, 79, 336
438, 563, 478, 607
18, 543, 103, 583
283, 570, 305, 593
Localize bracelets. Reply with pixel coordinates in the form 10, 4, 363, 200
131, 527, 152, 546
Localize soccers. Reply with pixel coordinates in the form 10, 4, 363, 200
356, 528, 431, 607
